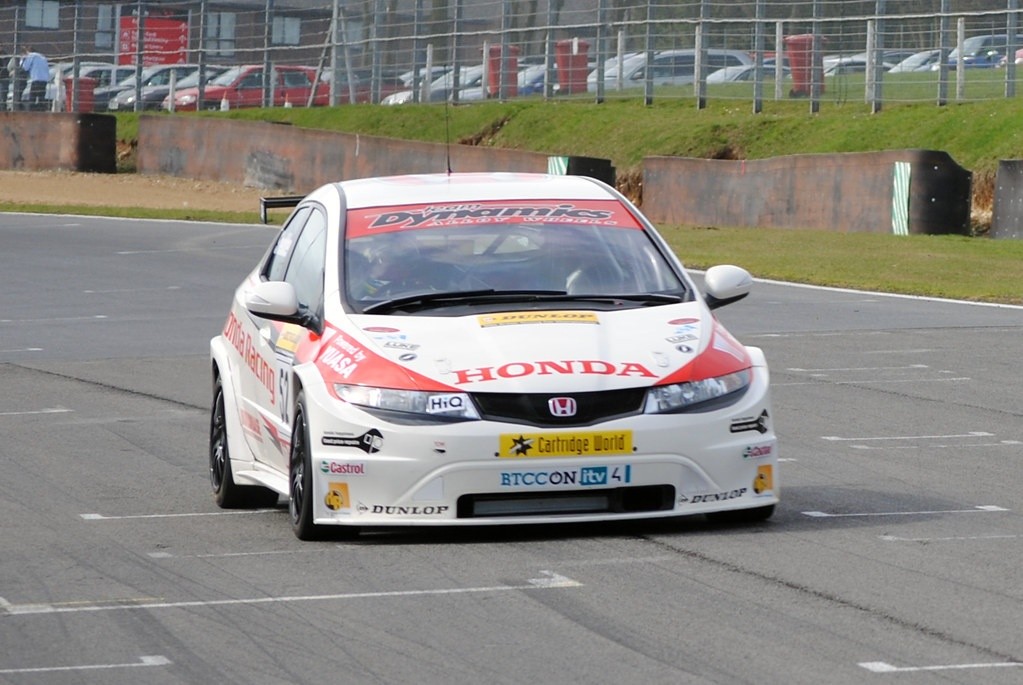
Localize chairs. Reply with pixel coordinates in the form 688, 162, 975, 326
531, 237, 614, 289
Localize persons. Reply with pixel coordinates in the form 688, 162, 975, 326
0, 45, 51, 112
349, 237, 426, 301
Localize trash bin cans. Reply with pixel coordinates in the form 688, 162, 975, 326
553, 38, 590, 94
488, 42, 523, 99
784, 34, 829, 92
62, 76, 97, 115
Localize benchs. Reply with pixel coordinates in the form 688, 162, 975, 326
258, 196, 306, 224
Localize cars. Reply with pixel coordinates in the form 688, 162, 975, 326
204, 163, 781, 543
0, 31, 1022, 114
